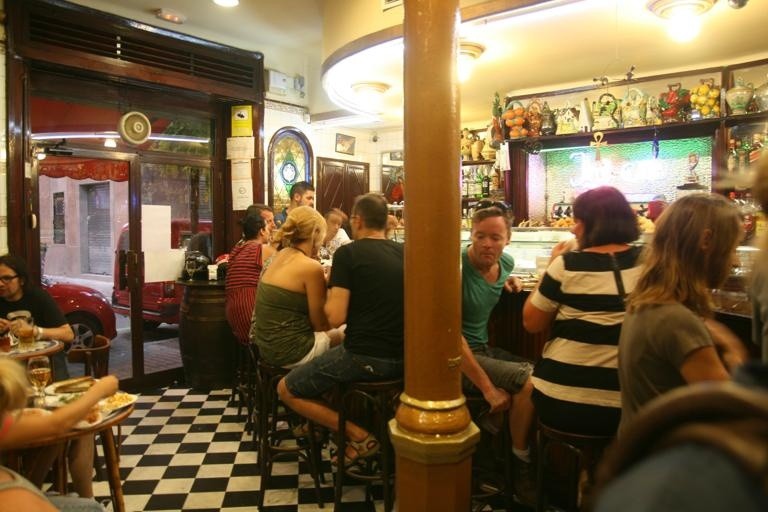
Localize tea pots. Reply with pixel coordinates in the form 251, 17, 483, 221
526, 100, 555, 137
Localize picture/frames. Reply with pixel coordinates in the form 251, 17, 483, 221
335, 134, 355, 155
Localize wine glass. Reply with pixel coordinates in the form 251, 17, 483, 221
185, 261, 196, 282
27, 356, 51, 409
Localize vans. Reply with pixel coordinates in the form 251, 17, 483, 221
108, 217, 213, 331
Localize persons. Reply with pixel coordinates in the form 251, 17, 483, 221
223, 181, 404, 468
524, 186, 767, 510
0, 254, 76, 382
460, 193, 535, 463
0, 466, 106, 512
0, 357, 120, 501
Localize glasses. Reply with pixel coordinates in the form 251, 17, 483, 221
476, 201, 508, 211
0, 275, 18, 284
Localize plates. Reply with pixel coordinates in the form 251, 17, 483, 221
43, 378, 137, 430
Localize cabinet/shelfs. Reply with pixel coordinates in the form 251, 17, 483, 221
461, 158, 506, 222
389, 226, 760, 321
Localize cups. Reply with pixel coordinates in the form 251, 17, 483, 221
15, 317, 35, 351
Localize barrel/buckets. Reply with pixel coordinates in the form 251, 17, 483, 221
177, 287, 241, 391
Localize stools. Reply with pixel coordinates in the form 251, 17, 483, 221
460, 390, 522, 510
534, 421, 613, 511
219, 317, 404, 511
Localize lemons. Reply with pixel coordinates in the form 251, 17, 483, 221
690, 84, 721, 115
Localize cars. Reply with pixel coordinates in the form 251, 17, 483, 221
40, 275, 117, 363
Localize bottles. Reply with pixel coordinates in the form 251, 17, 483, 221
462, 168, 499, 198
728, 191, 761, 246
461, 208, 472, 231
726, 133, 767, 178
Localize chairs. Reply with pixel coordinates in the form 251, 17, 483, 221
65, 334, 113, 479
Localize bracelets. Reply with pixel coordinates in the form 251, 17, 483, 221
29, 324, 36, 339
35, 324, 45, 342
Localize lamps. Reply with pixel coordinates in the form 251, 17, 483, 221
350, 82, 393, 107
458, 39, 487, 85
644, 0, 721, 47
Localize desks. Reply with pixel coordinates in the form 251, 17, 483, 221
173, 277, 237, 389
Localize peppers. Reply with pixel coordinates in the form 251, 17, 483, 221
660, 90, 689, 116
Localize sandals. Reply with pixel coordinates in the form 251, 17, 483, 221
331, 434, 380, 467
292, 424, 324, 440
